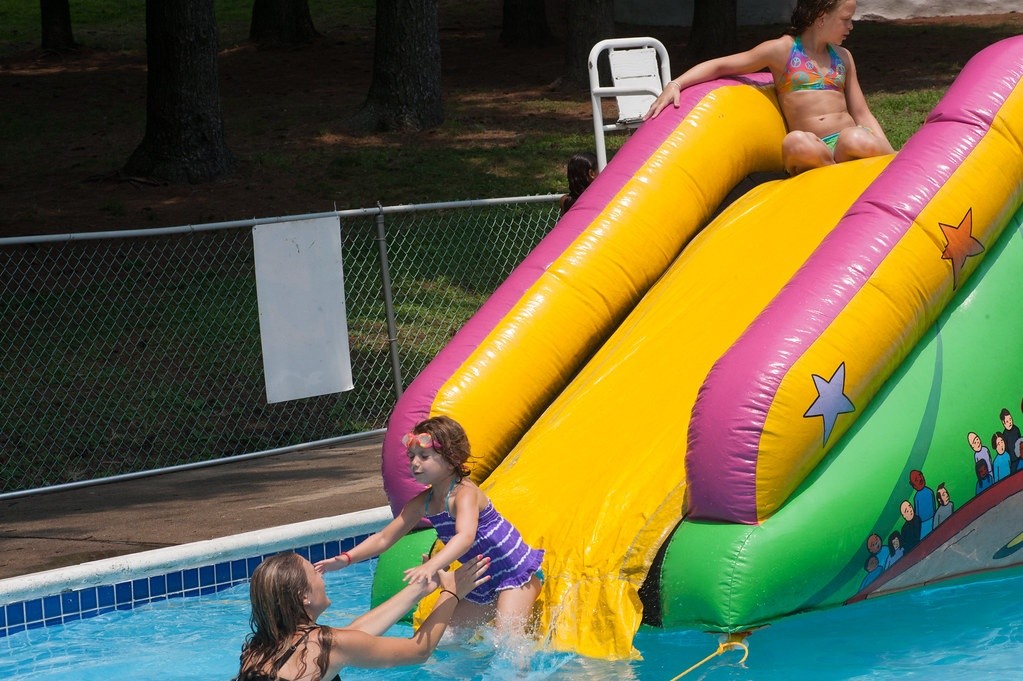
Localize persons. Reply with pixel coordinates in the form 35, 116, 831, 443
231, 552, 492, 681
559, 152, 599, 217
313, 415, 544, 632
643, 0, 896, 176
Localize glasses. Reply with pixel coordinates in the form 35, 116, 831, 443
403, 432, 443, 450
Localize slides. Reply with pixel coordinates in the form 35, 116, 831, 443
369, 33, 1023, 631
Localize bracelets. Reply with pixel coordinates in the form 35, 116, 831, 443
440, 589, 459, 603
341, 552, 351, 564
668, 81, 681, 88
